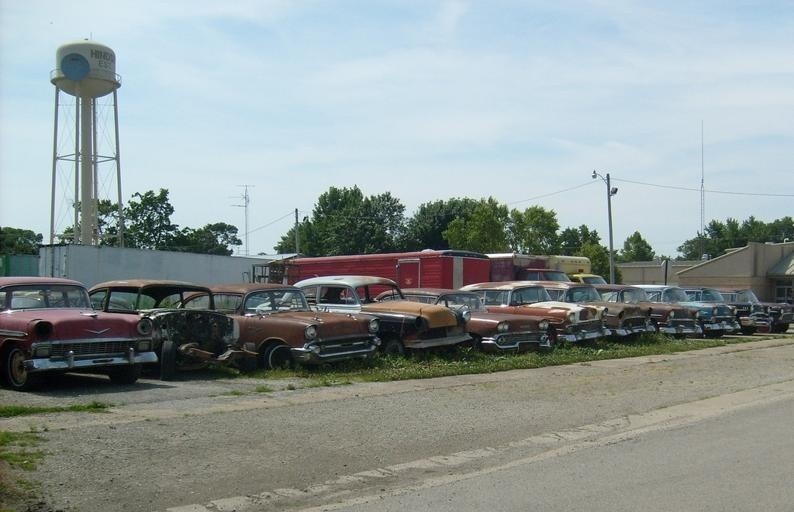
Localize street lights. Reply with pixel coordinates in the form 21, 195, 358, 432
592, 170, 614, 284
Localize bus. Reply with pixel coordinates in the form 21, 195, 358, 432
241, 247, 491, 298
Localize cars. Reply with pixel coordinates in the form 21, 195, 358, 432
75, 277, 260, 381
0, 274, 159, 392
176, 281, 382, 373
373, 287, 558, 356
255, 274, 473, 357
459, 248, 794, 347
